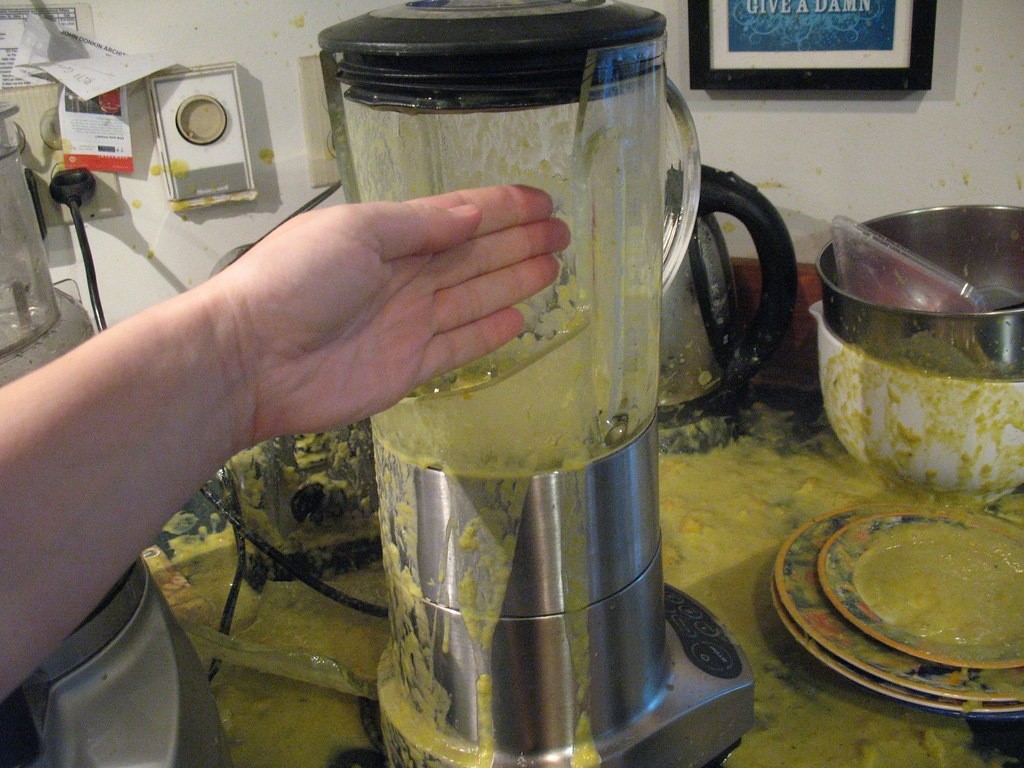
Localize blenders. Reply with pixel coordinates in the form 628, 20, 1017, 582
317, 0, 755, 766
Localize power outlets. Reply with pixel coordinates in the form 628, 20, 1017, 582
0, 85, 121, 229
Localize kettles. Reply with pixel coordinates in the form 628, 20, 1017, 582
659, 163, 798, 453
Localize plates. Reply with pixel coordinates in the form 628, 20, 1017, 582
771, 504, 1023, 719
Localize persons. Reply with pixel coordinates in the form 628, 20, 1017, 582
0, 183, 570, 702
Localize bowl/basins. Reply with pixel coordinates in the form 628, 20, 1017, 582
815, 202, 1024, 382
809, 299, 1024, 507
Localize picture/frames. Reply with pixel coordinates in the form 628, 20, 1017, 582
687, 0, 938, 91
